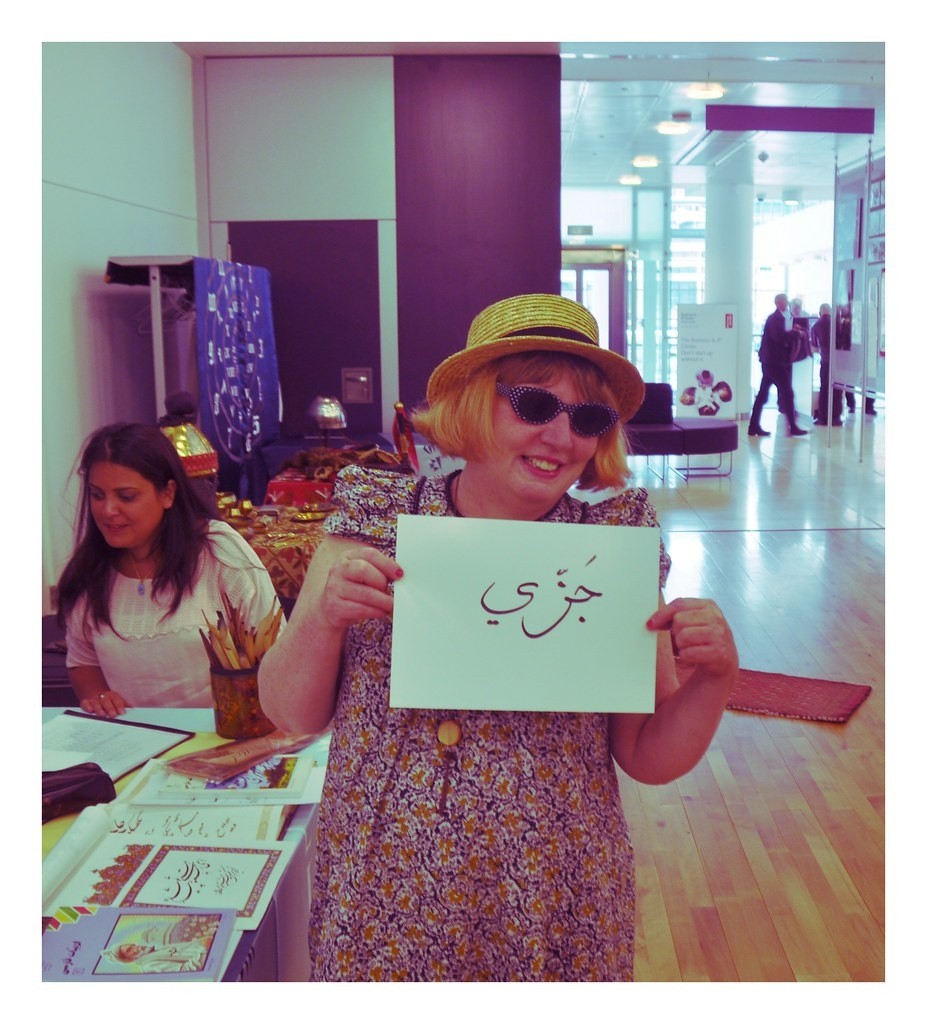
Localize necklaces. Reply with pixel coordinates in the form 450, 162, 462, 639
455, 474, 460, 509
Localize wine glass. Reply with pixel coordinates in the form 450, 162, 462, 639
238, 498, 252, 517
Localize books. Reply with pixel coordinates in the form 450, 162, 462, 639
159, 754, 315, 799
42, 906, 237, 982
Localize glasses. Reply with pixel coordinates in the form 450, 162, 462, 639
494, 380, 620, 436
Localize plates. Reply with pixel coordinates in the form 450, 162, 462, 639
299, 502, 337, 512
291, 512, 328, 521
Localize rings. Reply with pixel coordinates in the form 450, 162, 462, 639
98, 694, 105, 699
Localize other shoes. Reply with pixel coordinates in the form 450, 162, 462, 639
849, 408, 855, 413
814, 419, 827, 425
866, 407, 876, 416
748, 425, 770, 436
790, 426, 807, 435
832, 419, 842, 426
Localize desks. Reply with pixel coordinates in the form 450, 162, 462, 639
42, 698, 332, 982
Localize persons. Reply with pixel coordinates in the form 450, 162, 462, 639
811, 303, 878, 426
257, 294, 737, 982
747, 293, 808, 437
784, 298, 810, 331
57, 424, 287, 718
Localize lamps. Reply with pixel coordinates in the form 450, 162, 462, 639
685, 82, 724, 98
619, 175, 643, 185
658, 121, 690, 135
633, 156, 658, 167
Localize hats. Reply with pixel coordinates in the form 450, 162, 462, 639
426, 293, 646, 426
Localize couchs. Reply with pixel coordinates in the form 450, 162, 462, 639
621, 382, 738, 481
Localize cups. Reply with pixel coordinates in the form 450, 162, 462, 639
208, 663, 277, 740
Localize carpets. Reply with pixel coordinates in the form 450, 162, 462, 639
672, 657, 872, 725
